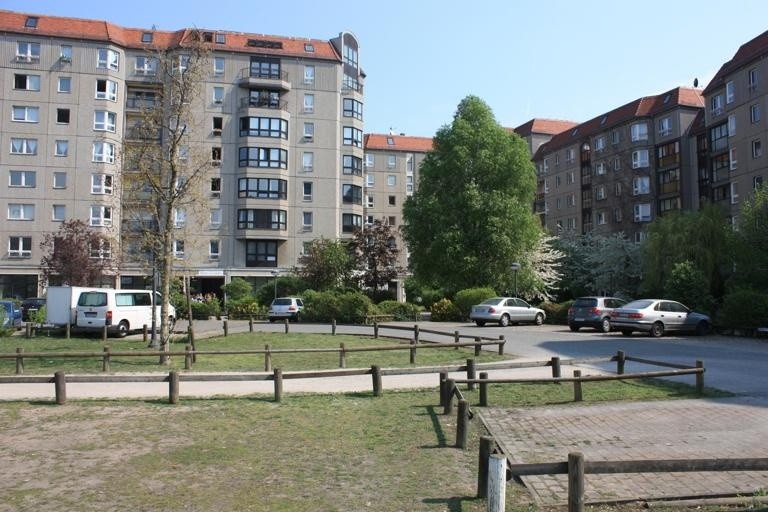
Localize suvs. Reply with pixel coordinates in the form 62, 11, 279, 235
567, 294, 629, 332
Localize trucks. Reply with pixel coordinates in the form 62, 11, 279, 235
45, 286, 98, 328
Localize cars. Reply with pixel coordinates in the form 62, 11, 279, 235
609, 297, 712, 336
470, 296, 546, 326
267, 297, 303, 322
0, 298, 45, 331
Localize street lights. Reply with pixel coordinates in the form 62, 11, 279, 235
269, 269, 279, 299
509, 263, 521, 299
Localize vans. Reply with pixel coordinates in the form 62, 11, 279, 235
75, 289, 176, 337
